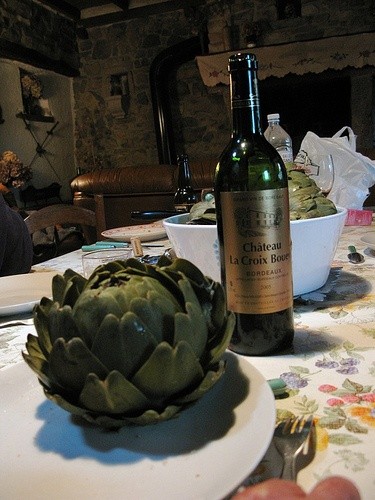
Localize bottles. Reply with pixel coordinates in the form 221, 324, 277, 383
172, 155, 198, 214
212, 52, 294, 357
263, 114, 292, 164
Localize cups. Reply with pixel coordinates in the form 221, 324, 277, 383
82, 250, 131, 280
304, 154, 334, 199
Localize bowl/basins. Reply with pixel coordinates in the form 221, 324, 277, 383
163, 206, 348, 297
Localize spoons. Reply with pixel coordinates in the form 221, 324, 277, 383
347, 246, 364, 264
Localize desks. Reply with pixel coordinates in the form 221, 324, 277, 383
0, 217, 375, 500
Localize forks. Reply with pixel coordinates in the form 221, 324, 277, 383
274, 414, 314, 480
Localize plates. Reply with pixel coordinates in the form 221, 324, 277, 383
100, 224, 167, 243
0, 271, 66, 317
0, 350, 276, 500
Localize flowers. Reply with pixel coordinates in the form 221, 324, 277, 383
0, 150, 33, 193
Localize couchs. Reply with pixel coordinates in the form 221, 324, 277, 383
70, 157, 219, 244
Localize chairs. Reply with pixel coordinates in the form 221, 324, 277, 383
23, 191, 105, 260
